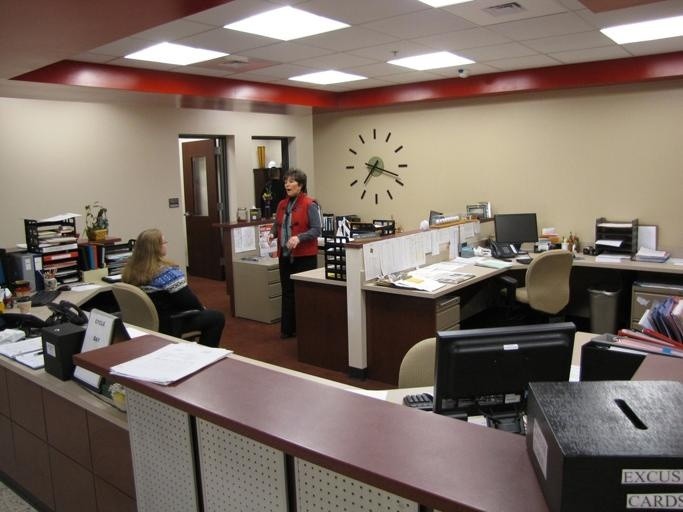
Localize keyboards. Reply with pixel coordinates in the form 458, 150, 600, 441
30, 291, 60, 306
516, 258, 533, 264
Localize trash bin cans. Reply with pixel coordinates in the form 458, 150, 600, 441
586, 285, 624, 335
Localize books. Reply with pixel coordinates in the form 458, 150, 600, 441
594, 254, 632, 263
578, 291, 682, 381
435, 271, 476, 284
633, 252, 671, 263
634, 246, 667, 259
0, 212, 133, 390
473, 259, 512, 270
107, 342, 236, 387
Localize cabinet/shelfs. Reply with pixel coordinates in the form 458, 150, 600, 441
252, 167, 281, 206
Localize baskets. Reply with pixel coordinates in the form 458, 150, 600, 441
85, 206, 108, 240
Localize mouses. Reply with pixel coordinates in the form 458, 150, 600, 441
57, 286, 71, 291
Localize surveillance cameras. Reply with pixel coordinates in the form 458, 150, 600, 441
457, 69, 464, 75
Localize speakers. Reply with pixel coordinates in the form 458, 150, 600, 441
41, 322, 86, 381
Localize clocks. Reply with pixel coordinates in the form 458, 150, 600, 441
341, 126, 408, 206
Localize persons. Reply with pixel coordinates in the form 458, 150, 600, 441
121, 227, 226, 348
266, 167, 323, 339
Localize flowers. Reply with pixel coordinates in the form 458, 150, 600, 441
261, 187, 272, 202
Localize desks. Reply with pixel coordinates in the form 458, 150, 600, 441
218, 213, 352, 325
0, 244, 681, 511
288, 216, 682, 383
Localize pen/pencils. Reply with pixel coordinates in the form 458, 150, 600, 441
38, 268, 55, 279
568, 232, 579, 243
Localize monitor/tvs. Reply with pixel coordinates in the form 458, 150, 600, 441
494, 213, 538, 254
433, 322, 576, 435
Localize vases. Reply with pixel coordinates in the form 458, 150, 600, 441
264, 203, 271, 220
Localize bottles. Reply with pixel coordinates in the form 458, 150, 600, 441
236, 207, 248, 223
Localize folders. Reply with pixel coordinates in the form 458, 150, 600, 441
645, 298, 682, 343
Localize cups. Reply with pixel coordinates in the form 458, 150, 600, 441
560, 242, 569, 251
43, 277, 57, 291
16, 301, 32, 313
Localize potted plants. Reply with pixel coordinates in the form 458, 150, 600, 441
81, 202, 109, 241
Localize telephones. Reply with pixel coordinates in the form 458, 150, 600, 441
47, 300, 88, 326
488, 239, 517, 259
